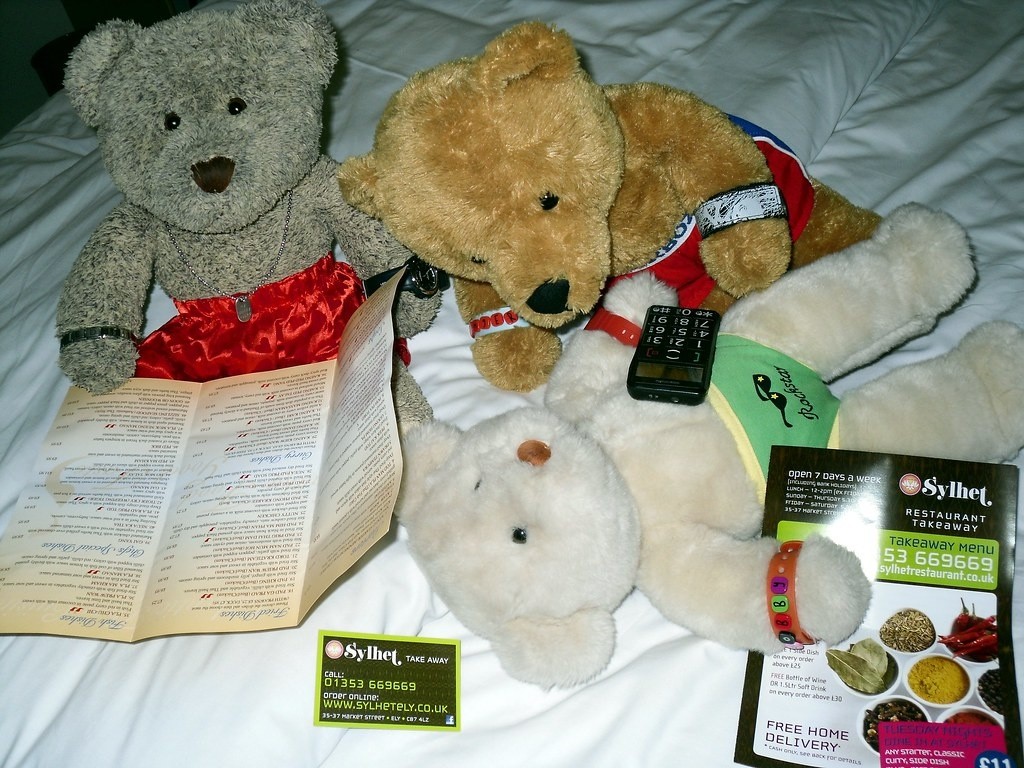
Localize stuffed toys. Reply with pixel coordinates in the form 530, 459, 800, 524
336, 19, 881, 390
57, 0, 443, 433
392, 206, 1024, 689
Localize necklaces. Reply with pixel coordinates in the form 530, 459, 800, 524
163, 191, 293, 323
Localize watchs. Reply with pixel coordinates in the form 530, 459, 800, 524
583, 306, 641, 347
362, 256, 450, 302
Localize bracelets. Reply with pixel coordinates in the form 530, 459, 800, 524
59, 326, 138, 350
693, 182, 789, 239
468, 306, 530, 339
768, 540, 821, 649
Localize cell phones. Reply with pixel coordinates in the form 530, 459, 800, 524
624, 298, 719, 407
